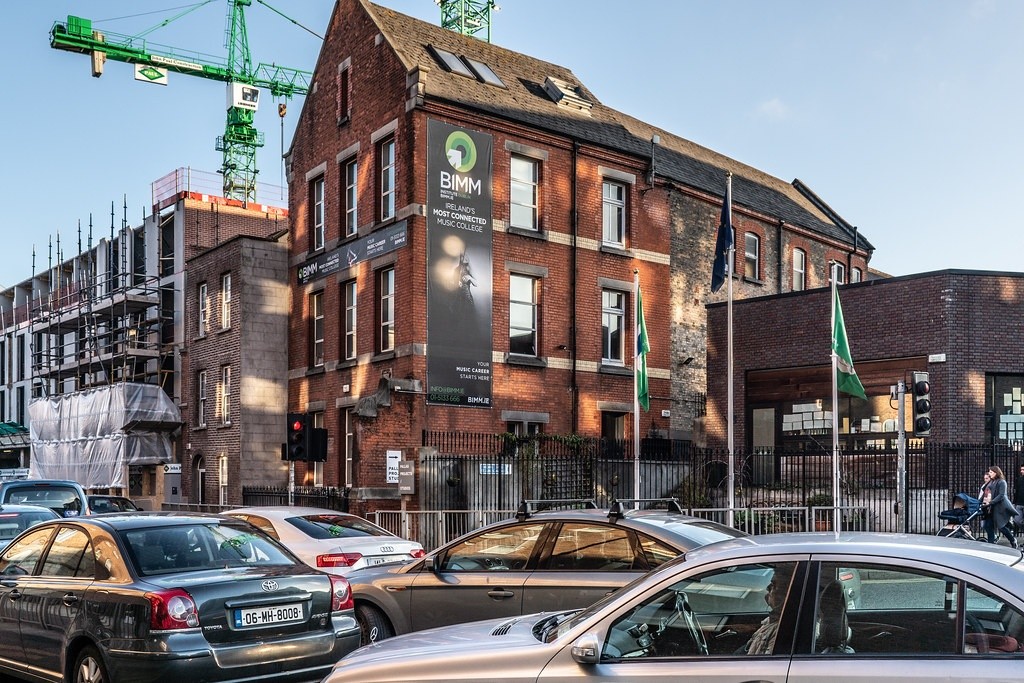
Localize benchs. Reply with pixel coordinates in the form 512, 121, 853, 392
965, 603, 1024, 654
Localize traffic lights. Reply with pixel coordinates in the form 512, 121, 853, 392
312, 428, 327, 462
286, 413, 308, 460
913, 372, 932, 436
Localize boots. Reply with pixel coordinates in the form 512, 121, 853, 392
1011, 536, 1019, 549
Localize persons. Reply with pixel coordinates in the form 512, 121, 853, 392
978, 472, 1000, 544
980, 466, 1019, 548
432, 252, 479, 329
1012, 464, 1024, 547
745, 563, 796, 656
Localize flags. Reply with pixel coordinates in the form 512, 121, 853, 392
711, 186, 733, 294
832, 287, 868, 401
637, 286, 651, 413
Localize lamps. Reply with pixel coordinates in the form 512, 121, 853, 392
640, 134, 661, 195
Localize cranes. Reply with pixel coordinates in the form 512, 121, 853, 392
48, 0, 331, 206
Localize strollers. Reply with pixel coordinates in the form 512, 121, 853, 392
935, 492, 989, 544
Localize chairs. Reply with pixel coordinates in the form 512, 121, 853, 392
131, 530, 252, 573
816, 580, 856, 654
550, 534, 633, 569
0, 517, 42, 536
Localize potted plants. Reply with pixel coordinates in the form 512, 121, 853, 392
684, 474, 882, 536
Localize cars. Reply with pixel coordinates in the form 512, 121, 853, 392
319, 531, 1024, 683
86, 495, 144, 516
0, 510, 361, 683
341, 499, 861, 646
219, 506, 426, 578
0, 504, 64, 553
0, 479, 90, 518
